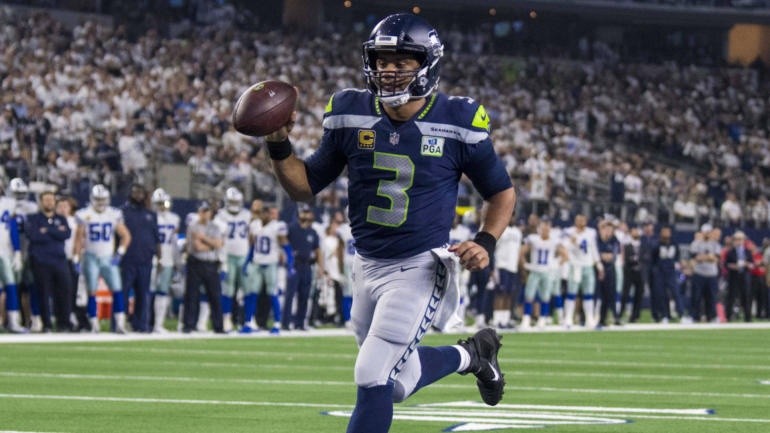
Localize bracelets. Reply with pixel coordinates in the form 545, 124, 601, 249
473, 232, 497, 257
266, 136, 292, 160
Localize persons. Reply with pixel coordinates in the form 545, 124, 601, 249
265, 14, 517, 433
0, 0, 770, 334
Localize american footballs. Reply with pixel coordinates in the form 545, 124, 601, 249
232, 79, 296, 137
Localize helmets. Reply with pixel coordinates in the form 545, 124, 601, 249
224, 188, 243, 214
8, 179, 27, 205
361, 15, 445, 106
150, 188, 170, 215
89, 185, 110, 212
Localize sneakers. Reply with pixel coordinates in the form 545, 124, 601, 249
455, 328, 504, 407
